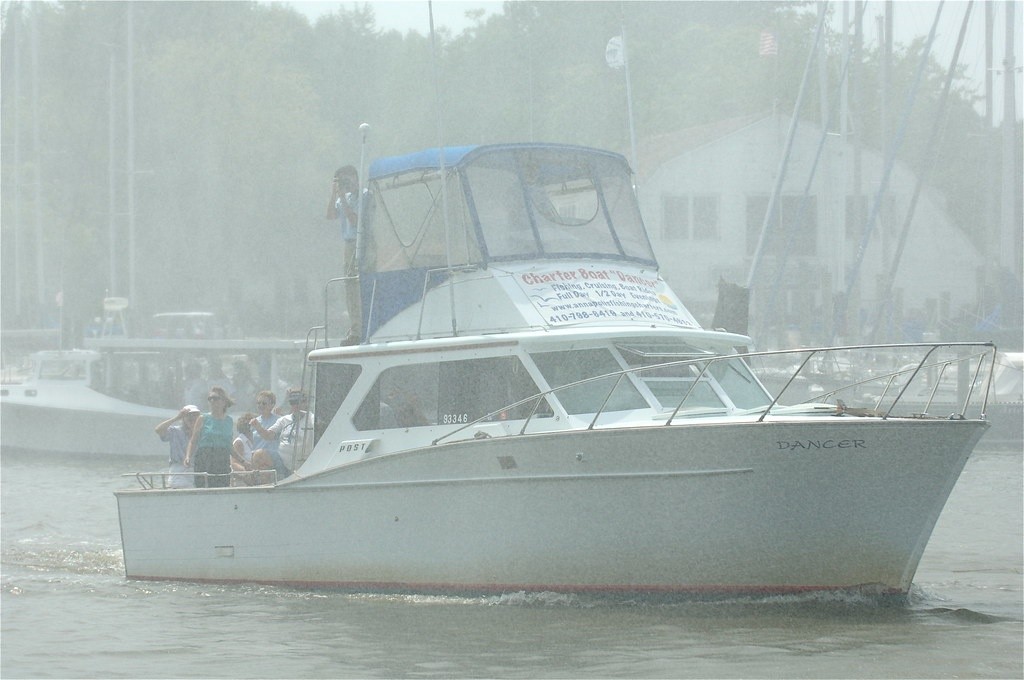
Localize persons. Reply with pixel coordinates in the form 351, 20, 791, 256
327, 165, 380, 348
154, 386, 314, 489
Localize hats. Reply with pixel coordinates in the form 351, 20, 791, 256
183, 405, 200, 412
286, 391, 306, 401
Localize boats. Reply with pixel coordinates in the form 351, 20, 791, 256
115, 1, 999, 604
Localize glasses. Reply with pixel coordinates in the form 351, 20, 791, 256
207, 396, 220, 401
256, 400, 267, 405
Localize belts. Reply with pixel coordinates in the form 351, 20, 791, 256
344, 238, 356, 243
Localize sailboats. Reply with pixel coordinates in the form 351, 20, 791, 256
0, 0, 220, 454
733, 0, 1024, 441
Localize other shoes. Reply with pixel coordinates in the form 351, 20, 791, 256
340, 335, 361, 346
